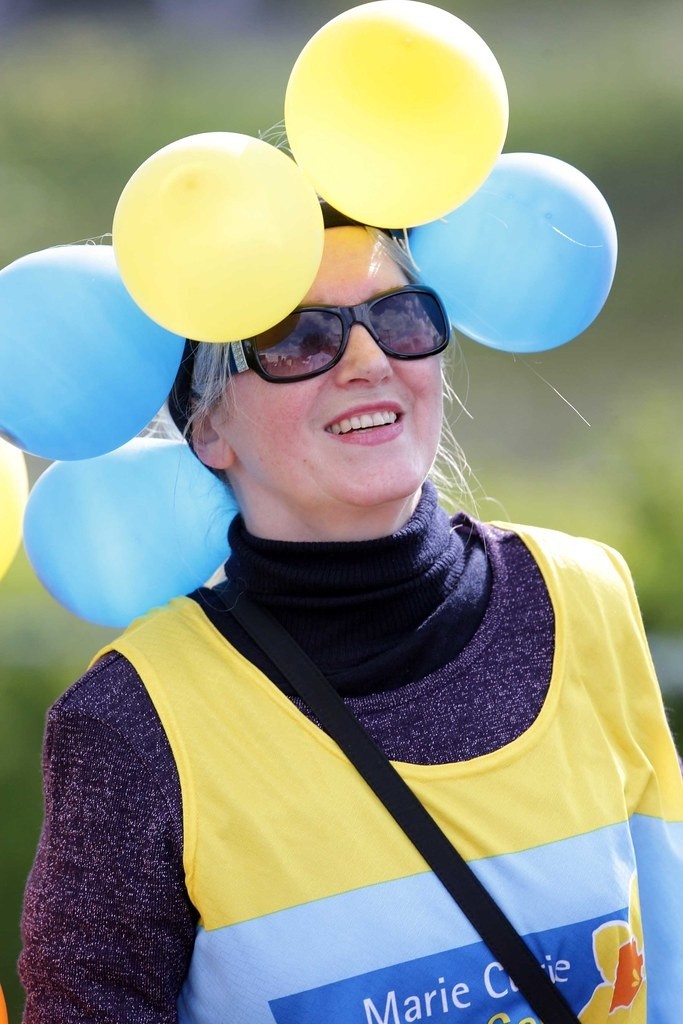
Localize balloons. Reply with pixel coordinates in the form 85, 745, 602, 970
21, 435, 243, 630
109, 130, 326, 344
0, 244, 188, 462
404, 151, 620, 355
282, 1, 510, 230
0, 435, 32, 584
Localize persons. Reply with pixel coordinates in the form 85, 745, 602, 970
17, 189, 683, 1023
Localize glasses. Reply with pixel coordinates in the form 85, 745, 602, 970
227, 285, 454, 386
